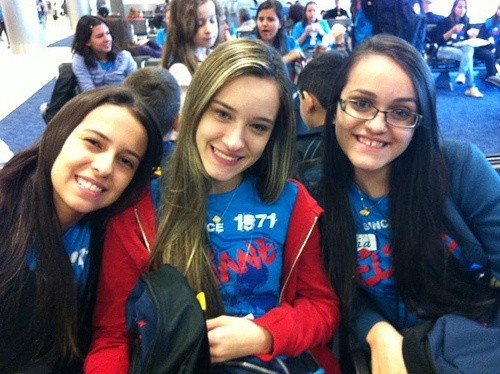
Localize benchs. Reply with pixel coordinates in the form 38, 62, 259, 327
424, 23, 487, 92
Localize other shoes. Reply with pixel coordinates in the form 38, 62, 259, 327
456, 73, 466, 85
466, 88, 485, 97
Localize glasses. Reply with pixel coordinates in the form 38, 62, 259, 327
335, 96, 424, 129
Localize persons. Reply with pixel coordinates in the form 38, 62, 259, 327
84, 38, 344, 374
288, 48, 353, 192
120, 66, 184, 218
300, 33, 500, 374
1, 85, 163, 374
1, 0, 500, 102
161, 0, 225, 183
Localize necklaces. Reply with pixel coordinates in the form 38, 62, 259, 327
204, 186, 238, 225
355, 183, 390, 217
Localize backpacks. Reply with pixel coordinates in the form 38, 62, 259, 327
124, 264, 212, 374
